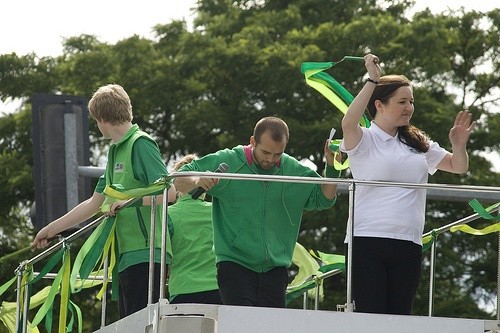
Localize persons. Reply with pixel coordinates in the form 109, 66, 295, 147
341, 54, 476, 315
173, 116, 343, 308
167, 153, 222, 305
30, 84, 176, 320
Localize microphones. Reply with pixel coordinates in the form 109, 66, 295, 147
191, 163, 230, 200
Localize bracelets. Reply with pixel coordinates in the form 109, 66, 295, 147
365, 78, 378, 85
